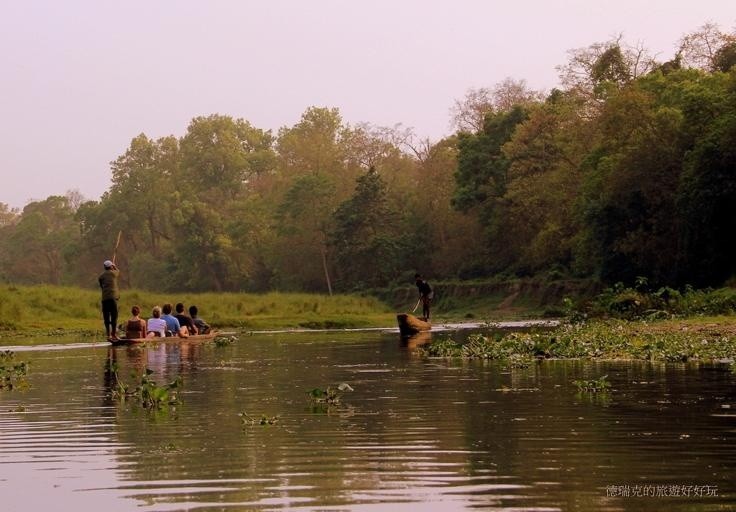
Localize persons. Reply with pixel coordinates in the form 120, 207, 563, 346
126, 303, 209, 339
98, 260, 121, 341
416, 277, 434, 320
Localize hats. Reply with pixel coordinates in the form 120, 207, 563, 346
103, 261, 115, 267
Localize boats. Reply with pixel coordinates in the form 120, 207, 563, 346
109, 331, 221, 344
395, 312, 431, 331
401, 331, 431, 349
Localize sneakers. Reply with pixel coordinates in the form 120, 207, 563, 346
106, 336, 120, 340
